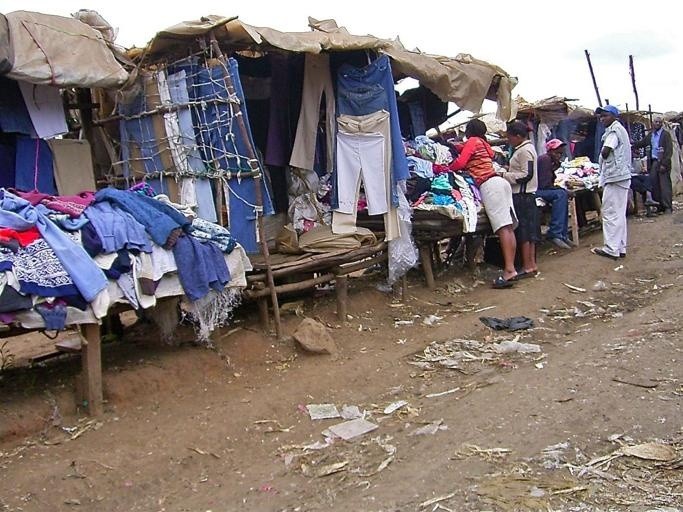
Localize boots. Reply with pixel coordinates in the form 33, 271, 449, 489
645, 200, 659, 205
647, 213, 656, 217
551, 238, 576, 249
594, 248, 625, 261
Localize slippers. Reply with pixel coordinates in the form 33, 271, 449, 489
492, 276, 517, 288
518, 268, 537, 279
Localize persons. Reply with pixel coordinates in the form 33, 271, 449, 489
594, 104, 632, 261
629, 117, 673, 217
432, 118, 577, 288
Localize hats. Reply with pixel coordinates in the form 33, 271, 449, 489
596, 105, 618, 117
546, 139, 566, 151
